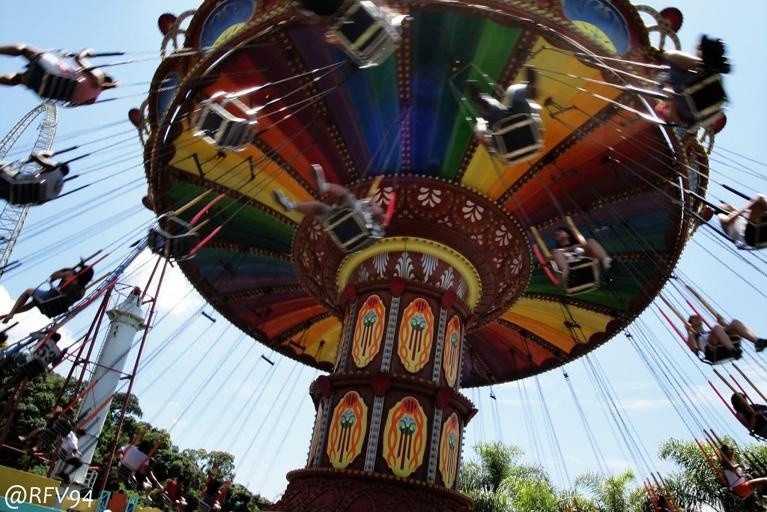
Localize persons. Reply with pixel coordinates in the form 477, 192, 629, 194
0, 42, 114, 106
464, 62, 540, 150
15, 406, 74, 450
135, 469, 154, 502
162, 473, 189, 508
272, 161, 386, 247
0, 330, 69, 385
116, 436, 154, 489
197, 479, 223, 512
730, 390, 766, 440
656, 494, 675, 512
709, 192, 766, 249
653, 33, 733, 130
0, 149, 69, 205
55, 427, 86, 485
719, 445, 766, 498
685, 312, 767, 362
0, 256, 94, 325
546, 224, 617, 292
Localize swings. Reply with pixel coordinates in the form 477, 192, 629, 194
321, 92, 419, 253
1, 38, 308, 511
444, 44, 766, 511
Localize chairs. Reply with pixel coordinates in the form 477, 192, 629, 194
0, 1, 401, 512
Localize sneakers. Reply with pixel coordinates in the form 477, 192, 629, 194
754, 337, 766, 352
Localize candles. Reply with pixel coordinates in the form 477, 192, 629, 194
566, 185, 643, 274
